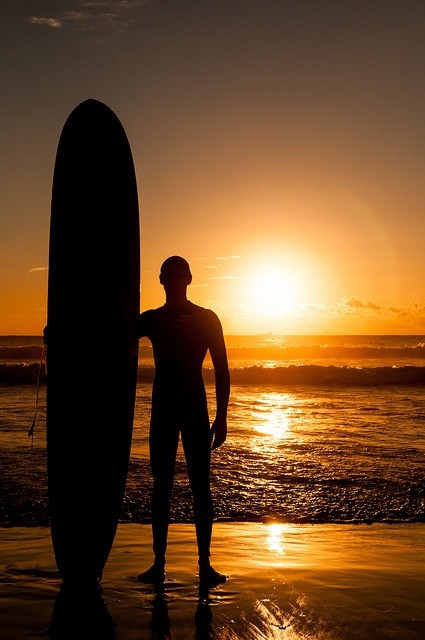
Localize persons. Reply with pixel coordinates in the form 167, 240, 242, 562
44, 256, 230, 585
